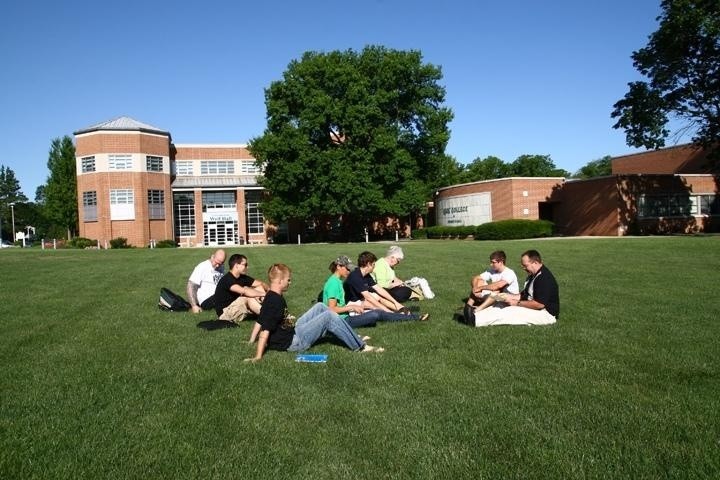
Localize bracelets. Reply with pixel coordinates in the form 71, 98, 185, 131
517, 299, 522, 306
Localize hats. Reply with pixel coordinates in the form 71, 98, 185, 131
335, 255, 358, 269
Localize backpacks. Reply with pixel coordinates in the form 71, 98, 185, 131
158, 288, 192, 311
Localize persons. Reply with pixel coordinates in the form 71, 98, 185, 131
462, 249, 561, 329
373, 244, 412, 303
465, 250, 520, 313
344, 251, 413, 319
214, 252, 296, 326
322, 255, 431, 327
239, 263, 387, 364
185, 249, 228, 313
